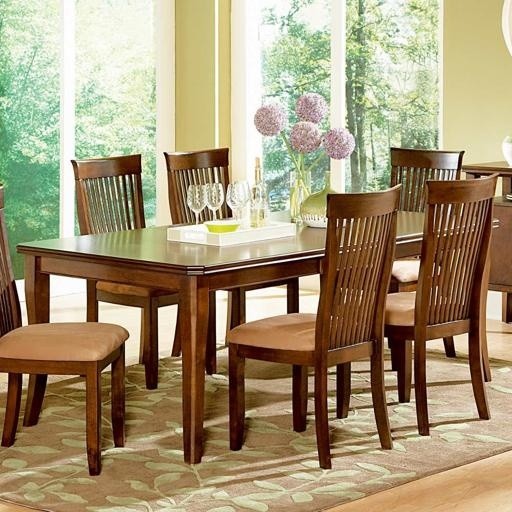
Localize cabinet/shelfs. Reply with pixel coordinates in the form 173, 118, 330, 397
461, 162, 512, 322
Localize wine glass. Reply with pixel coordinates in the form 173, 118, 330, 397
205, 183, 224, 221
187, 185, 208, 230
225, 180, 251, 230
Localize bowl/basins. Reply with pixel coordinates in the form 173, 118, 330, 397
203, 220, 242, 233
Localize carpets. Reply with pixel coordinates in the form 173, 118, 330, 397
0, 341, 511, 512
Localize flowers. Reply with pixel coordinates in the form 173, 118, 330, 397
253, 92, 356, 220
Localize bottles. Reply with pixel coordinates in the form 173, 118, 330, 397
250, 157, 269, 228
